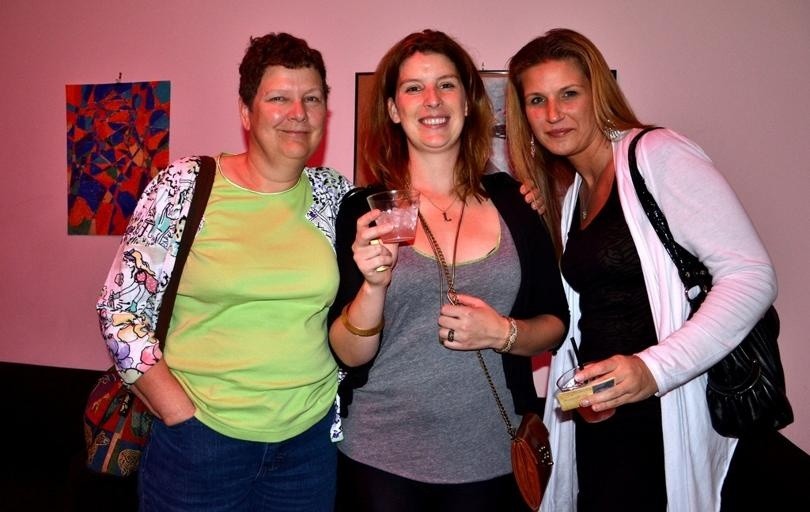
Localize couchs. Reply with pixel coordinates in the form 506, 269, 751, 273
0, 359, 151, 512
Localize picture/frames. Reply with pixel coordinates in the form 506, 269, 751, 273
353, 72, 615, 186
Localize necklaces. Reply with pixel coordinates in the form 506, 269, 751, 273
579, 165, 603, 220
409, 186, 469, 346
414, 187, 459, 222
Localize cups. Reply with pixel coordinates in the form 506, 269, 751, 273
556, 362, 617, 424
367, 188, 421, 247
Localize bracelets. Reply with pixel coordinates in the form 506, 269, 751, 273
494, 313, 518, 354
340, 303, 386, 337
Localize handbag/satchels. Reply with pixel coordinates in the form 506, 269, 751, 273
83, 357, 152, 476
683, 259, 797, 439
512, 412, 554, 511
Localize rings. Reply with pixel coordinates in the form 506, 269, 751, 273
447, 331, 454, 342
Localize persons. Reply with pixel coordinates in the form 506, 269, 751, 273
93, 32, 358, 512
326, 27, 570, 511
502, 27, 779, 512
